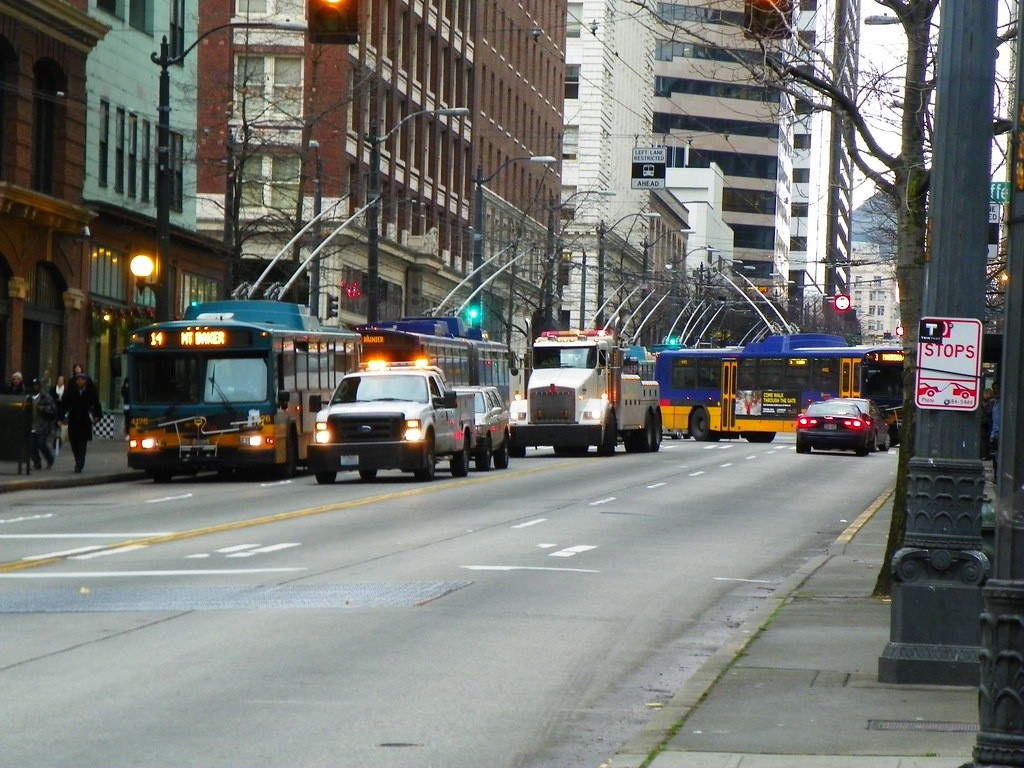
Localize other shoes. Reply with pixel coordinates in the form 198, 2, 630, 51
46, 456, 55, 469
33, 458, 42, 469
74, 461, 85, 472
125, 434, 129, 441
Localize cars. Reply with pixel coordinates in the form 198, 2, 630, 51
795, 401, 879, 456
825, 398, 891, 452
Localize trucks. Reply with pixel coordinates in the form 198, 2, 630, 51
509, 330, 663, 458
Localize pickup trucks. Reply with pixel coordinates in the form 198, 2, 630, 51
307, 360, 477, 484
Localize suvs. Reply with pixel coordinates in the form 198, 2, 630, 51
450, 385, 511, 472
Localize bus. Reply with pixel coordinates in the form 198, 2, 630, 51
654, 333, 905, 446
350, 317, 511, 418
127, 300, 364, 482
621, 344, 688, 380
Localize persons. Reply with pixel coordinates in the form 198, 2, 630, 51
8, 363, 104, 474
982, 382, 1001, 485
122, 377, 132, 442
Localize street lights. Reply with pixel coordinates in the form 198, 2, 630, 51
669, 228, 742, 338
639, 229, 696, 347
368, 108, 470, 324
545, 191, 616, 331
471, 156, 557, 327
596, 212, 661, 328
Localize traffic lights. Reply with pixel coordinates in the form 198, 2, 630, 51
896, 326, 904, 336
305, 0, 359, 45
834, 295, 851, 313
744, 1, 793, 40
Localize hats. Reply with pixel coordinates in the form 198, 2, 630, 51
29, 378, 42, 386
75, 373, 87, 379
12, 372, 23, 381
